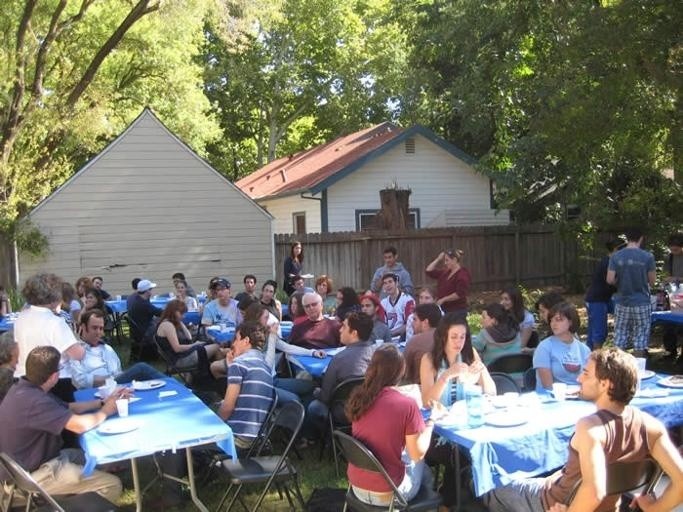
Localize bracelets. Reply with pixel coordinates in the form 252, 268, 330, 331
424, 417, 437, 424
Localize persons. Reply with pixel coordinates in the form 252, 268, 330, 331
585, 232, 615, 350
57, 274, 82, 338
423, 248, 475, 316
477, 347, 683, 512
282, 240, 305, 294
210, 298, 281, 379
531, 293, 564, 343
472, 302, 524, 365
532, 296, 580, 342
313, 276, 342, 316
245, 306, 323, 402
344, 344, 451, 509
530, 304, 590, 396
333, 283, 361, 319
66, 310, 170, 388
377, 270, 413, 342
127, 277, 141, 308
308, 311, 381, 421
157, 319, 277, 512
0, 332, 20, 404
235, 274, 257, 309
153, 301, 222, 374
77, 289, 111, 324
604, 225, 659, 360
289, 293, 342, 350
314, 275, 337, 314
127, 280, 165, 341
288, 277, 316, 296
170, 281, 197, 310
368, 246, 414, 300
91, 275, 111, 302
75, 278, 91, 300
0, 285, 12, 319
403, 305, 448, 381
417, 314, 497, 512
405, 286, 446, 339
498, 289, 538, 350
172, 273, 195, 299
255, 280, 283, 325
357, 297, 387, 342
200, 278, 244, 326
289, 275, 317, 299
663, 232, 683, 360
288, 293, 309, 324
5, 271, 84, 449
200, 279, 218, 316
0, 347, 135, 503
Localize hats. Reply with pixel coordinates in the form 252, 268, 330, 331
137, 279, 157, 293
207, 277, 230, 290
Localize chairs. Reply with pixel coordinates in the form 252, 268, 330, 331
488, 353, 534, 374
490, 372, 521, 395
152, 333, 203, 383
105, 304, 122, 344
522, 367, 537, 389
1, 453, 120, 512
318, 376, 366, 478
331, 427, 443, 511
196, 387, 280, 510
215, 398, 307, 512
124, 315, 156, 363
566, 457, 661, 512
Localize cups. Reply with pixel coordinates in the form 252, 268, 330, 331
376, 339, 384, 348
116, 399, 128, 417
220, 324, 227, 332
553, 383, 566, 403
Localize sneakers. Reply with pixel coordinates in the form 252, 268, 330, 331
170, 471, 205, 497
113, 488, 143, 507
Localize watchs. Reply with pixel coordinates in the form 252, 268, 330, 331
0, 299, 8, 303
100, 396, 106, 408
311, 350, 317, 358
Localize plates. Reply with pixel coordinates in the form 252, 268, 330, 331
133, 380, 165, 390
642, 370, 655, 379
552, 385, 578, 399
98, 420, 142, 434
94, 392, 101, 396
657, 377, 683, 388
485, 413, 527, 427
